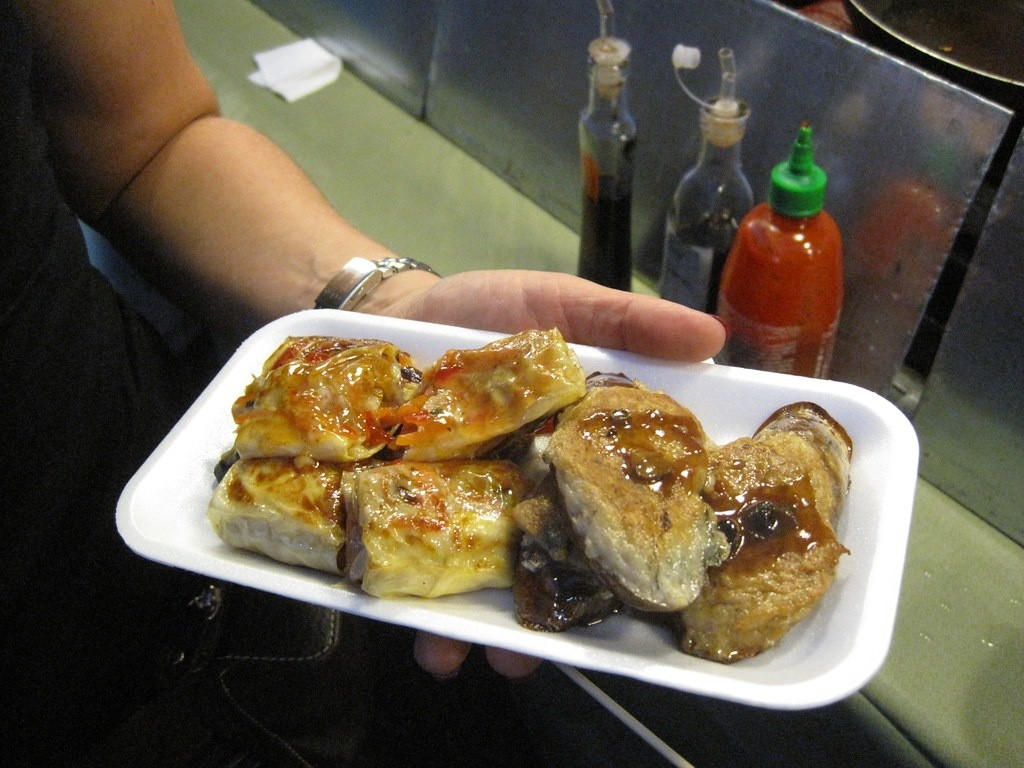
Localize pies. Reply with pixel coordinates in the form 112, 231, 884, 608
210, 326, 586, 596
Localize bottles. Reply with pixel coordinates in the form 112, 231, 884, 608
577, 2, 636, 293
715, 122, 842, 378
657, 46, 756, 317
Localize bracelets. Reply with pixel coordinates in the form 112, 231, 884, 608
312, 256, 442, 310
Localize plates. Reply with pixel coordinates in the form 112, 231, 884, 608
114, 309, 920, 713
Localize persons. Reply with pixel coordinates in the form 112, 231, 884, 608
1, 1, 730, 768
